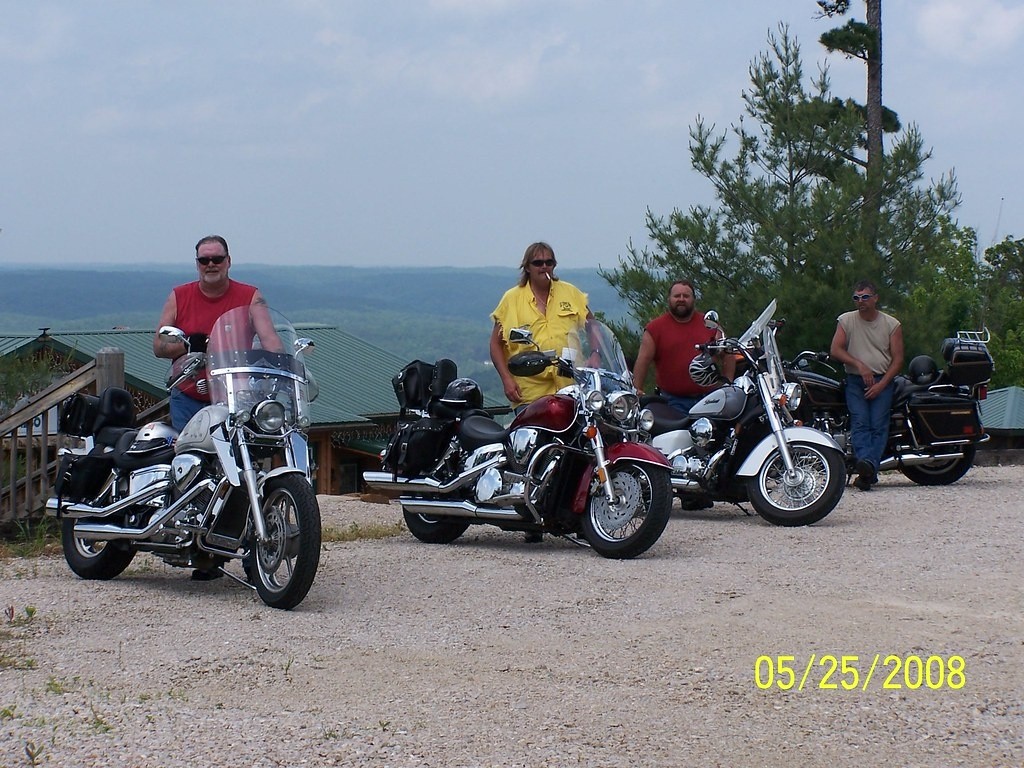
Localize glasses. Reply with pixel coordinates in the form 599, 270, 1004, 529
528, 259, 555, 266
852, 293, 876, 301
196, 254, 228, 265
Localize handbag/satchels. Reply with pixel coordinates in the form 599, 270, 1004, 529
391, 360, 456, 411
59, 391, 100, 438
381, 418, 445, 474
55, 452, 115, 500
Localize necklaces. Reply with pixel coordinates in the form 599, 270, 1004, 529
530, 285, 546, 306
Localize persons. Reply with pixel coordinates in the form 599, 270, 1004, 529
154, 238, 289, 582
489, 242, 602, 543
631, 281, 738, 510
830, 281, 904, 492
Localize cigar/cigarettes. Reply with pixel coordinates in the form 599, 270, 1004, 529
545, 271, 551, 280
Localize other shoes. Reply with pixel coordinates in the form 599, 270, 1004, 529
524, 530, 542, 543
856, 459, 878, 484
683, 497, 713, 510
854, 476, 870, 491
192, 567, 224, 581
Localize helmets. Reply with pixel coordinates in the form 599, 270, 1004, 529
689, 353, 720, 386
441, 377, 483, 408
908, 354, 937, 384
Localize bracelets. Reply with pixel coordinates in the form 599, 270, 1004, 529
589, 349, 602, 358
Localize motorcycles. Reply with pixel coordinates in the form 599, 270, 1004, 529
360, 318, 675, 561
46, 305, 322, 610
737, 299, 996, 486
636, 311, 847, 529
